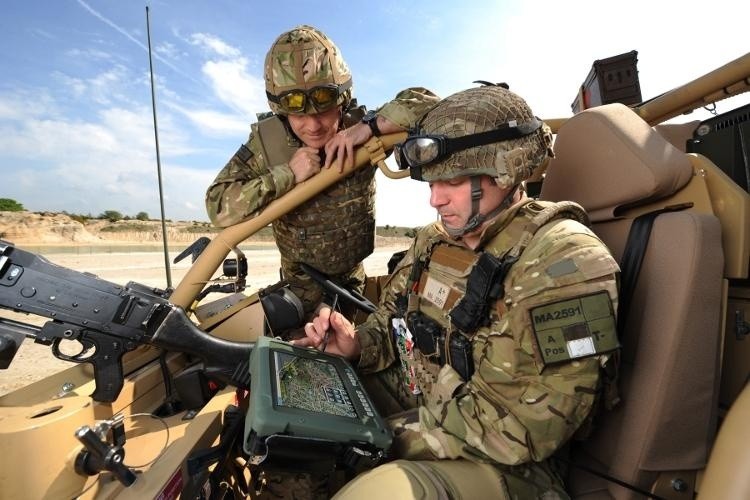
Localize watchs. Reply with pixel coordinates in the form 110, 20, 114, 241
363, 112, 383, 138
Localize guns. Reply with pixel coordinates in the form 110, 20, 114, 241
0, 239, 256, 401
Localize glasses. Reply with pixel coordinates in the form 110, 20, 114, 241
393, 116, 539, 170
266, 76, 353, 113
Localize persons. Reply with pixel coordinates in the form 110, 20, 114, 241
205, 26, 440, 342
293, 79, 623, 500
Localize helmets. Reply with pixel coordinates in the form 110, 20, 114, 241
263, 24, 352, 115
410, 85, 555, 190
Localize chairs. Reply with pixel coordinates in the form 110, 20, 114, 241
538, 104, 725, 500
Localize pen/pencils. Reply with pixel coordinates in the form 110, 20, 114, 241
323, 293, 339, 352
